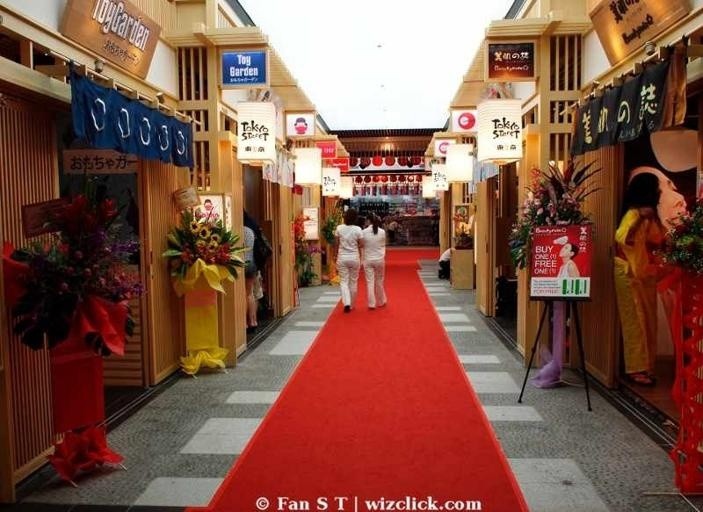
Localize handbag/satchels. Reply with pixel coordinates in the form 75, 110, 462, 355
252, 229, 273, 270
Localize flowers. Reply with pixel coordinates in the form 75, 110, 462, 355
652, 193, 703, 274
506, 153, 605, 270
294, 244, 325, 283
0, 192, 150, 357
161, 206, 251, 299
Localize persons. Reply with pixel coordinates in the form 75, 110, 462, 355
612, 171, 667, 386
335, 209, 365, 313
243, 210, 273, 334
382, 211, 400, 245
557, 242, 581, 278
362, 212, 388, 309
438, 248, 450, 279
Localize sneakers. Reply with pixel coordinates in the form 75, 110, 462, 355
438, 269, 444, 278
344, 306, 351, 311
369, 302, 386, 309
